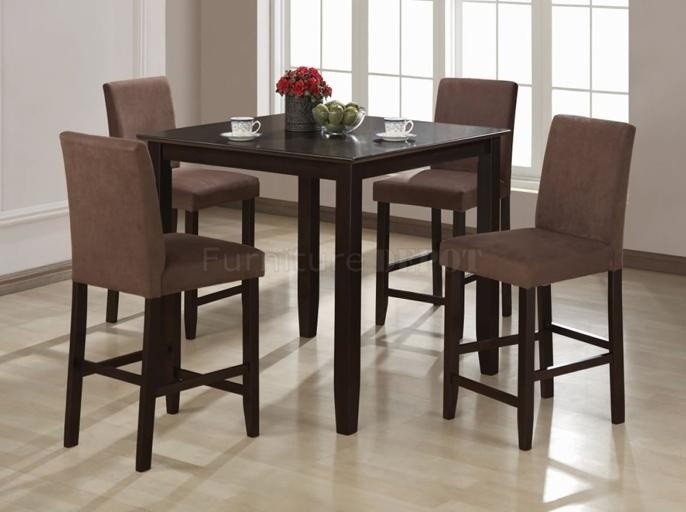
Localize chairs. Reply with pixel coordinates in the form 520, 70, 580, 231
437, 113, 637, 451
372, 77, 519, 340
58, 125, 266, 473
99, 75, 260, 340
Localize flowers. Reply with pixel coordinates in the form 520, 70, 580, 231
275, 65, 332, 103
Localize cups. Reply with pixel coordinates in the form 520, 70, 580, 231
230, 117, 261, 136
384, 117, 414, 137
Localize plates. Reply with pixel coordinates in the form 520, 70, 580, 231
377, 133, 417, 141
220, 132, 263, 141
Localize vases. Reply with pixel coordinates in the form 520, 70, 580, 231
284, 94, 323, 134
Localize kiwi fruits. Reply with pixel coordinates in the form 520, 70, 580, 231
314, 102, 360, 132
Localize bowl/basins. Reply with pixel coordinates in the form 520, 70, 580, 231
312, 108, 365, 135
